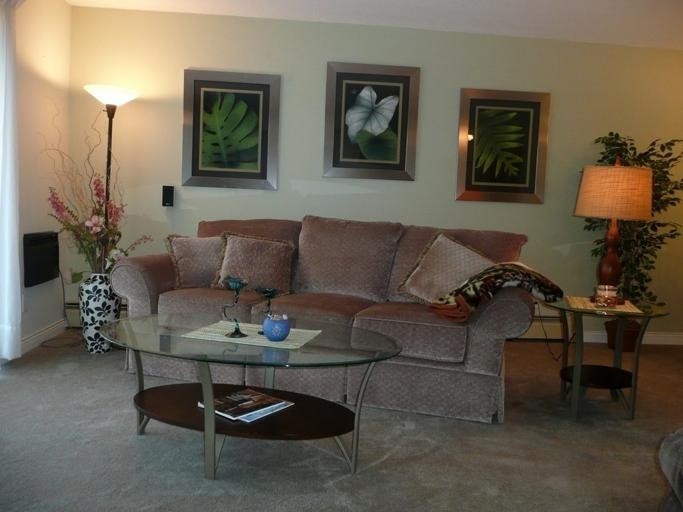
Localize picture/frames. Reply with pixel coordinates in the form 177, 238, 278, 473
321, 61, 421, 181
455, 87, 551, 204
180, 68, 282, 191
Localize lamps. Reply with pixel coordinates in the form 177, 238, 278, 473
83, 84, 139, 261
573, 151, 653, 306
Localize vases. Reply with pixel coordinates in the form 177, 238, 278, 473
78, 273, 122, 356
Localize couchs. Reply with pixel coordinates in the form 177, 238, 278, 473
110, 216, 535, 424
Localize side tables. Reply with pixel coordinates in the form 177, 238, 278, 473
541, 296, 675, 420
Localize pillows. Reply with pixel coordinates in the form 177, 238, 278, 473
164, 229, 297, 296
396, 230, 500, 306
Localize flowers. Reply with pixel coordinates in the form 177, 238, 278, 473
42, 105, 154, 285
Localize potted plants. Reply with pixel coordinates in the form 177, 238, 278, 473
574, 131, 683, 353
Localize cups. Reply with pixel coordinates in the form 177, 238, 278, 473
595, 285, 618, 309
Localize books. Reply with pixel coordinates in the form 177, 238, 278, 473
197, 388, 296, 422
